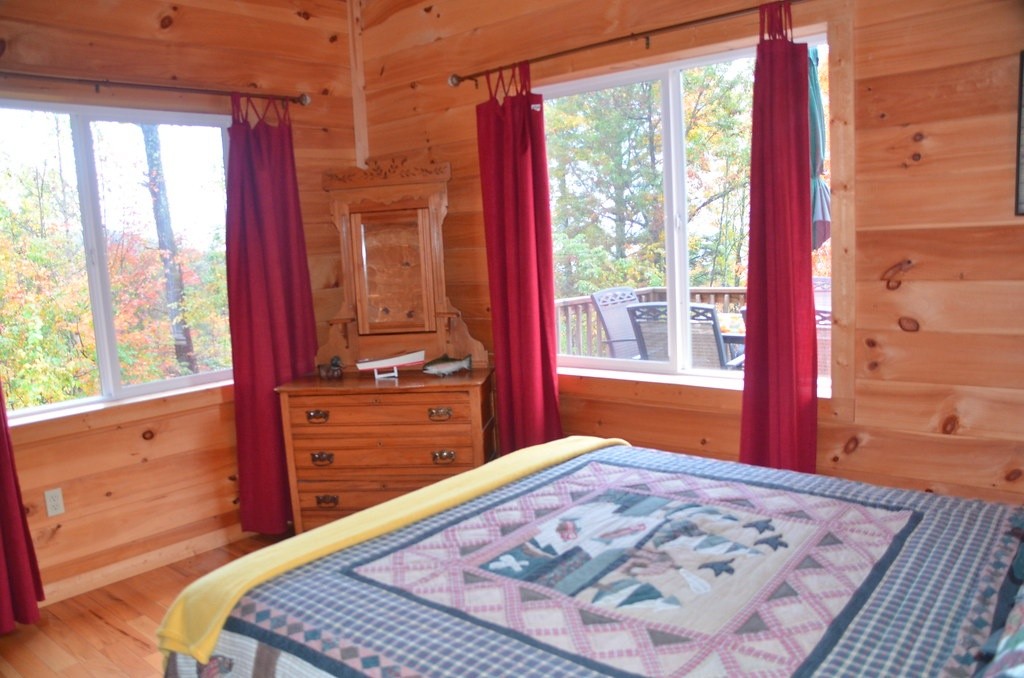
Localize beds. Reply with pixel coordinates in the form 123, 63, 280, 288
156, 432, 1024, 678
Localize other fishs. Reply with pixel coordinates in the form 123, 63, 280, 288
422, 353, 472, 378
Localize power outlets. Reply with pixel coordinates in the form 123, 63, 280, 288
45, 487, 65, 517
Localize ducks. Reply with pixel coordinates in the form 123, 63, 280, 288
317, 356, 346, 380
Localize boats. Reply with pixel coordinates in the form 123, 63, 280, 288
355, 350, 425, 372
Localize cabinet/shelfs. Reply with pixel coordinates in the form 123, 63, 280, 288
274, 151, 497, 538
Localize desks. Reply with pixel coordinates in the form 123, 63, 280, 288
715, 312, 745, 346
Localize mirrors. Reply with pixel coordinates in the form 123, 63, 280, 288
351, 207, 436, 335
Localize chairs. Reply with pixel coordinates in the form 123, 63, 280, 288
627, 300, 746, 372
813, 277, 831, 377
590, 285, 643, 360
